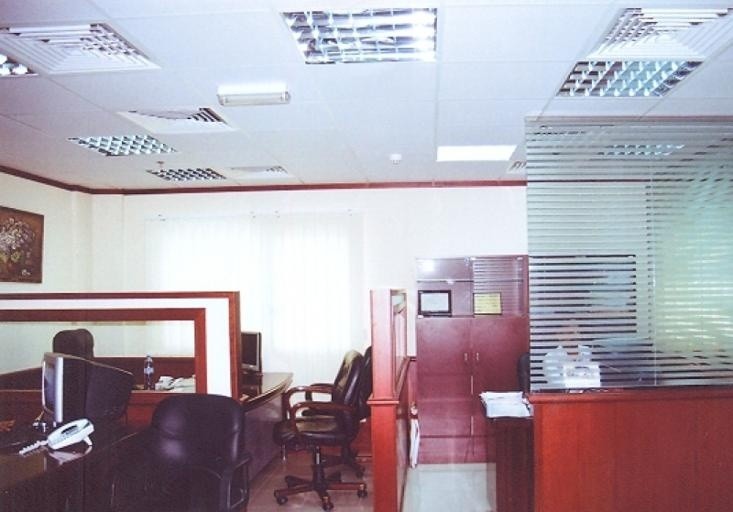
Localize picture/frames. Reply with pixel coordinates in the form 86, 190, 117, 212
0, 206, 45, 283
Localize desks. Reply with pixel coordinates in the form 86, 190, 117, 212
0, 422, 149, 512
155, 370, 294, 481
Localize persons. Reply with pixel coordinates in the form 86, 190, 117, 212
541, 320, 602, 387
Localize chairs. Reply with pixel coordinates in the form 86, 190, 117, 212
106, 392, 253, 512
51, 328, 93, 359
272, 344, 370, 511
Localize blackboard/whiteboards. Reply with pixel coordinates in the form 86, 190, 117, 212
45, 418, 96, 452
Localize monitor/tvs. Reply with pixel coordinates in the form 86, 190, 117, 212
240, 330, 261, 377
590, 336, 654, 382
41, 352, 135, 423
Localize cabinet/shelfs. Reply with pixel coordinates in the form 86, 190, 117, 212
413, 254, 638, 465
